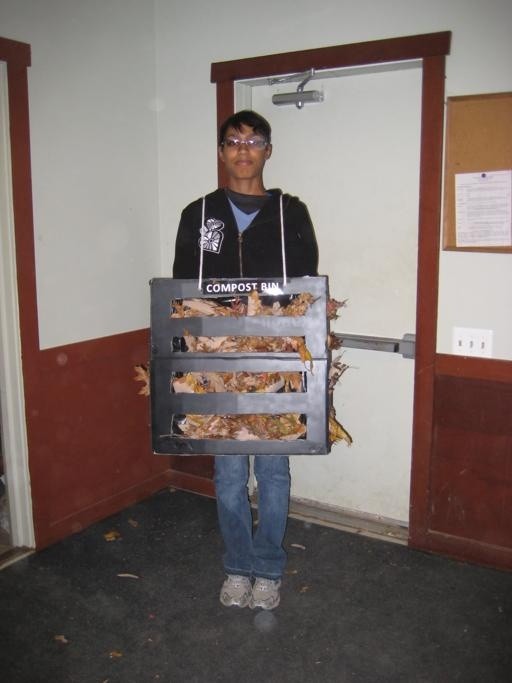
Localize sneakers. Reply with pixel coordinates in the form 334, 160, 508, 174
219, 571, 253, 610
248, 574, 282, 612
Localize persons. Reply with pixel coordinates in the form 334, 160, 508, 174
173, 109, 319, 608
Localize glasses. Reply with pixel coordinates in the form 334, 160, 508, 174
222, 137, 267, 149
